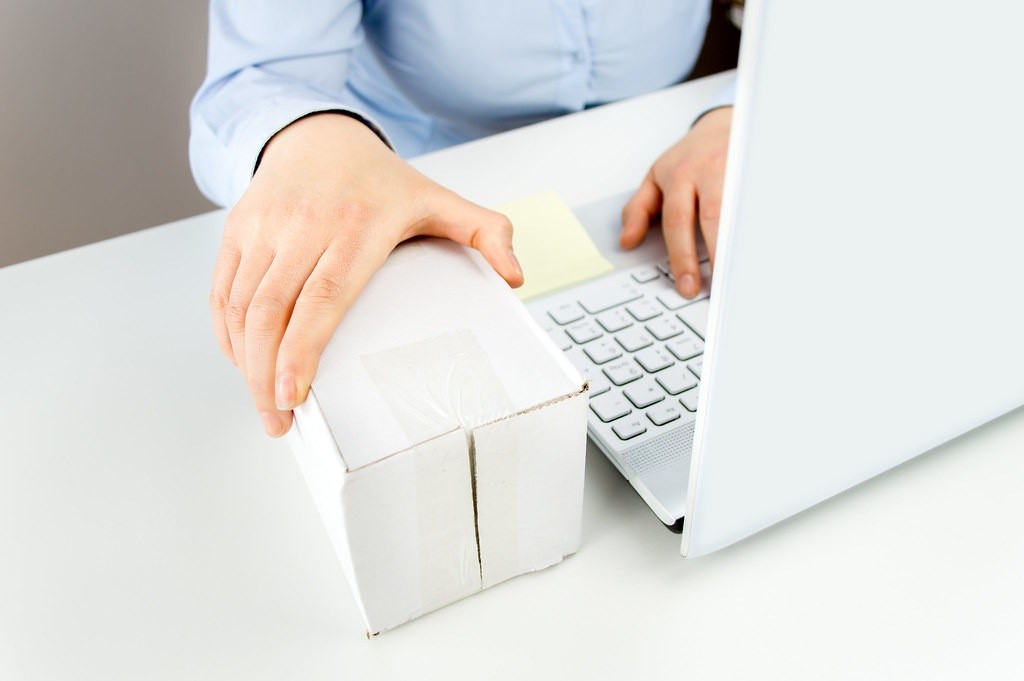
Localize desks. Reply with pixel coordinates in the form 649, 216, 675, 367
0, 71, 1024, 681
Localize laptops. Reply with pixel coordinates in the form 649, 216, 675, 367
520, 0, 1024, 556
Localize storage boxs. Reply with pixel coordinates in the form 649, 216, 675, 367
285, 236, 590, 642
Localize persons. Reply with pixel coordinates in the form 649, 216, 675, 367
189, 1, 737, 437
